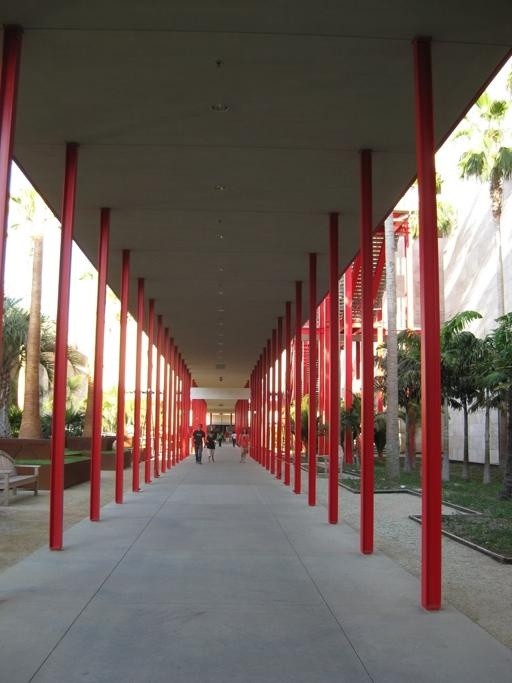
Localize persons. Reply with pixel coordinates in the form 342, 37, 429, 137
204, 430, 217, 463
239, 428, 250, 462
191, 423, 206, 464
218, 432, 222, 447
231, 431, 237, 447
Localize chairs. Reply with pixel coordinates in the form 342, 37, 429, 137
0, 450, 41, 507
316, 445, 344, 479
